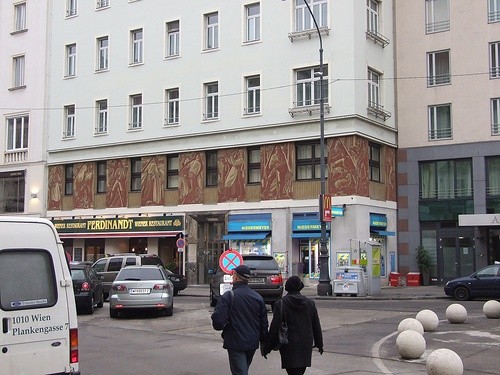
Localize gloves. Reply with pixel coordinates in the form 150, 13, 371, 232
260, 346, 264, 356
263, 347, 271, 359
312, 345, 323, 355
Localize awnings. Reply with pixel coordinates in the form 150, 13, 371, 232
224, 232, 269, 239
372, 230, 396, 236
291, 233, 329, 238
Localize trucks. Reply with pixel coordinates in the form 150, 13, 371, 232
0, 215, 81, 375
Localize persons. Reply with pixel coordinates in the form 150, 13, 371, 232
211, 265, 269, 375
268, 276, 324, 375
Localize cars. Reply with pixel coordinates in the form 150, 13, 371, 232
444, 264, 500, 300
70, 264, 105, 315
166, 269, 186, 298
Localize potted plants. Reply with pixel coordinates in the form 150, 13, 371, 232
416, 245, 435, 286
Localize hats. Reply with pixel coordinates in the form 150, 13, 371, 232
231, 265, 251, 279
284, 276, 304, 292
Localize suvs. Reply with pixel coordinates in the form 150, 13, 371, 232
91, 254, 165, 301
108, 263, 174, 319
208, 253, 283, 307
